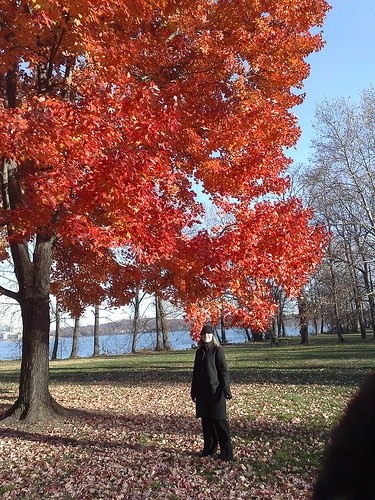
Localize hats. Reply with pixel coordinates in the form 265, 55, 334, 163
199, 325, 214, 335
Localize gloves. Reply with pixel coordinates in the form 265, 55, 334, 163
225, 392, 232, 400
192, 396, 196, 403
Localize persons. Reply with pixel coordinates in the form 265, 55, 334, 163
190, 325, 233, 461
